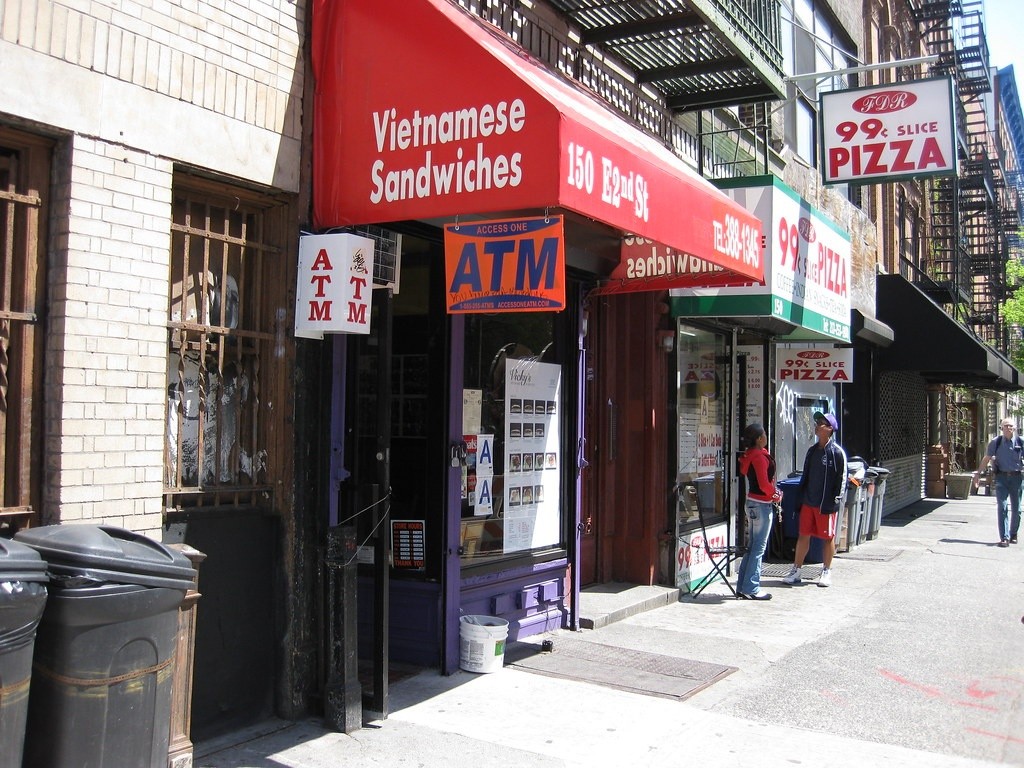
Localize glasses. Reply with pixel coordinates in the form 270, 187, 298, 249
814, 421, 829, 427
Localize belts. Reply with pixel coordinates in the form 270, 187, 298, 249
997, 470, 1021, 476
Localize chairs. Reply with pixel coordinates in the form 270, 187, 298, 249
689, 493, 748, 600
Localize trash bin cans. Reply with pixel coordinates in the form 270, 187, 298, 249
779, 478, 825, 563
11, 522, 200, 767
1, 532, 52, 768
833, 466, 889, 551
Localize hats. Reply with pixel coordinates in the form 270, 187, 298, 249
814, 412, 838, 431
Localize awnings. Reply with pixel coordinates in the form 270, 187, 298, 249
312, 0, 853, 348
876, 273, 1024, 391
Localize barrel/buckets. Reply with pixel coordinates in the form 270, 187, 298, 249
459, 615, 509, 673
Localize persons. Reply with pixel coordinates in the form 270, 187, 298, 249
973, 417, 1024, 546
740, 423, 780, 602
781, 411, 847, 587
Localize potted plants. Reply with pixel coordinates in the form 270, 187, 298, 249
945, 393, 975, 501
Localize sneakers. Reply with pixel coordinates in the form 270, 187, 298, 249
818, 568, 831, 587
998, 538, 1009, 547
743, 592, 772, 600
1010, 533, 1017, 543
782, 567, 801, 585
736, 591, 743, 597
